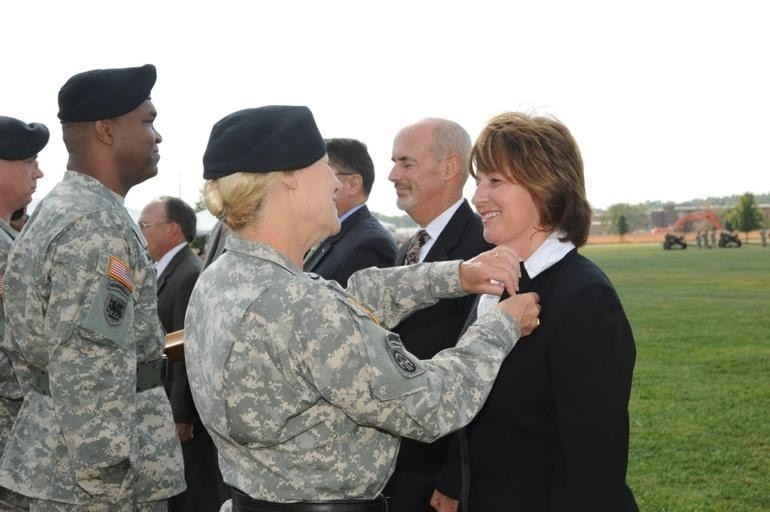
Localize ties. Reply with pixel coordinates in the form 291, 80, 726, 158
402, 230, 431, 266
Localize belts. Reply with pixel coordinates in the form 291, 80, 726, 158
31, 355, 168, 396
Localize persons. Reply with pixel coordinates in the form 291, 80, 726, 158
0, 62, 191, 511
0, 114, 50, 282
132, 195, 223, 512
450, 112, 644, 511
294, 135, 389, 281
694, 226, 719, 250
182, 103, 542, 512
759, 223, 770, 246
373, 114, 496, 512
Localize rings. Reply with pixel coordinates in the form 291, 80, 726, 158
537, 317, 540, 326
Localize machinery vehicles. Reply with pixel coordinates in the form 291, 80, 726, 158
651, 208, 730, 234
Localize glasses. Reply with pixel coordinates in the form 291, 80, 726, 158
139, 220, 173, 232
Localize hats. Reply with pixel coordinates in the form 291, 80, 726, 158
0, 115, 50, 160
57, 64, 157, 121
204, 106, 326, 179
